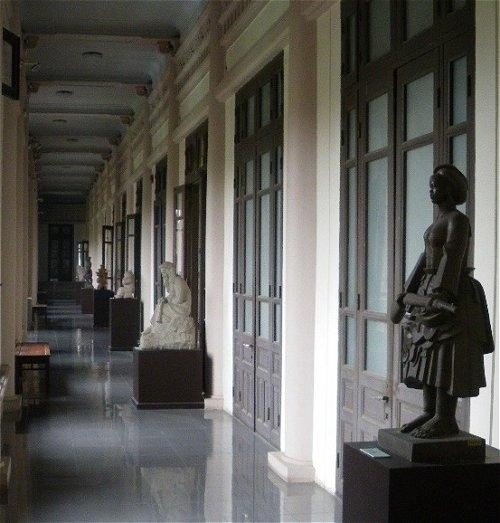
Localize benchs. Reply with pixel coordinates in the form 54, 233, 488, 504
16, 340, 51, 395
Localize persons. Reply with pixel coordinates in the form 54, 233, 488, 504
389, 162, 497, 441
141, 259, 193, 337
